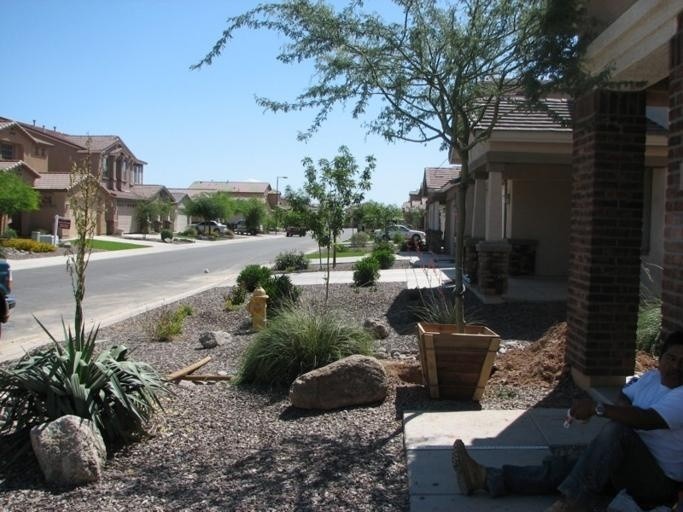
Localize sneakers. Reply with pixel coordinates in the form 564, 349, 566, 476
452, 439, 480, 496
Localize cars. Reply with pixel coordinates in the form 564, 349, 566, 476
373, 224, 426, 241
286, 223, 306, 237
195, 220, 260, 235
0, 254, 16, 323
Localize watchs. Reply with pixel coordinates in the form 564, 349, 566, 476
594, 400, 606, 418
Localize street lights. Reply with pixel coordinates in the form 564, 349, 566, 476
275, 176, 288, 236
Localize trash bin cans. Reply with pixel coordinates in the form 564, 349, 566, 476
508, 238, 538, 276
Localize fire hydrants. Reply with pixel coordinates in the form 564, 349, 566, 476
244, 282, 269, 332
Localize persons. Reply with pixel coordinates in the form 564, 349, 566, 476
449, 328, 683, 512
